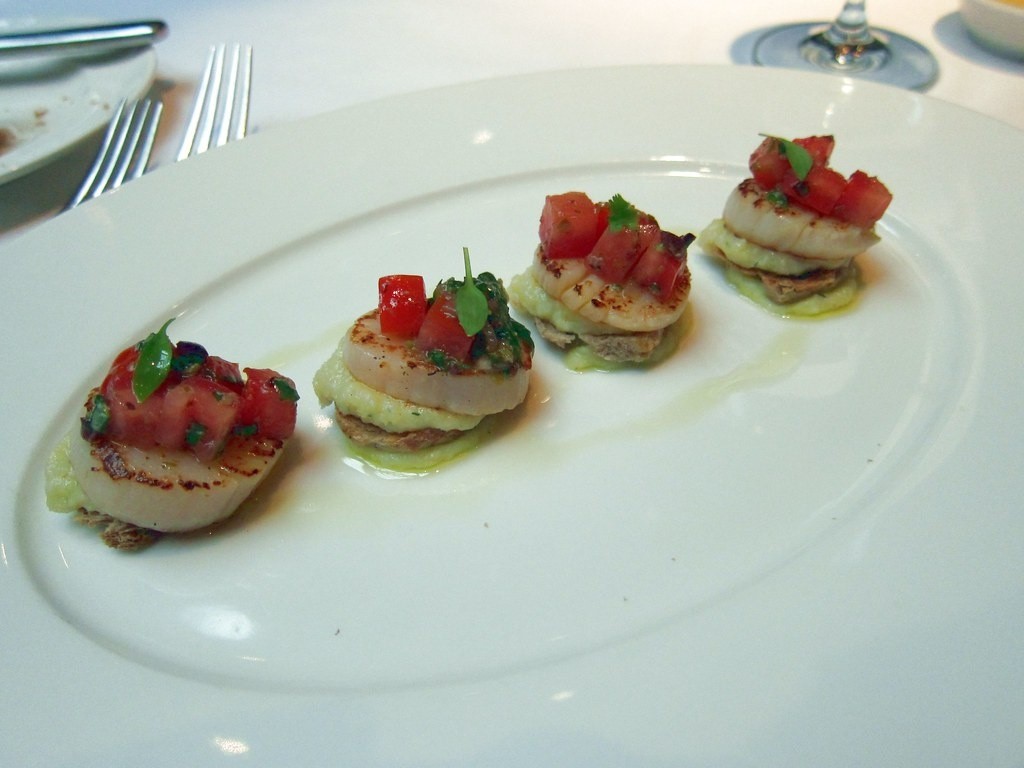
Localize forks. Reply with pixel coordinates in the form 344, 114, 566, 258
175, 44, 254, 160
55, 97, 165, 214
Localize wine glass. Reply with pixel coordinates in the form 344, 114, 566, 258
731, 0, 939, 92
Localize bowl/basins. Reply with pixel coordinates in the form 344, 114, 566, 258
959, 0, 1024, 57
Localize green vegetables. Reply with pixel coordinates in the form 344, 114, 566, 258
89, 340, 300, 445
456, 245, 488, 337
608, 194, 641, 232
132, 318, 177, 404
758, 133, 814, 181
425, 271, 534, 376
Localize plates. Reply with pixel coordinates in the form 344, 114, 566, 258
0, 62, 1024, 768
0, 18, 155, 186
